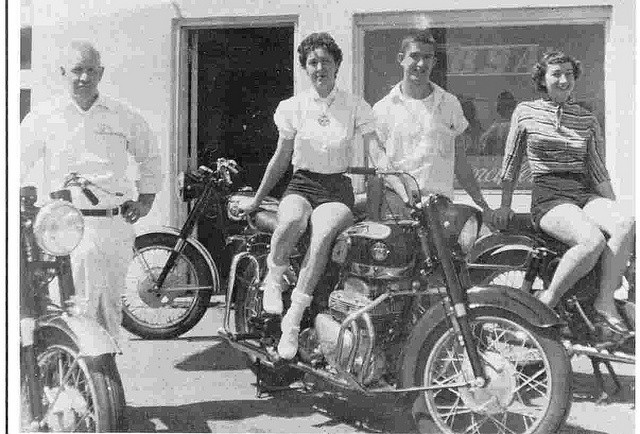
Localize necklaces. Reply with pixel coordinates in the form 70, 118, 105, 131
313, 87, 339, 126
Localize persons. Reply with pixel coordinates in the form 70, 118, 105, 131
20, 40, 162, 349
456, 93, 486, 155
479, 89, 517, 156
235, 31, 411, 361
488, 50, 636, 336
366, 30, 497, 232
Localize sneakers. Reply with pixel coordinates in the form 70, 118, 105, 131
263, 274, 285, 316
277, 316, 302, 360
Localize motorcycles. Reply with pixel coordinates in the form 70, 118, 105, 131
20, 174, 126, 433
121, 157, 367, 339
218, 167, 573, 433
466, 212, 636, 403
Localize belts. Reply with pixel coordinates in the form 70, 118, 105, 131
79, 205, 129, 217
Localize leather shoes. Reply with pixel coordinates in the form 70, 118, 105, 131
591, 304, 632, 336
